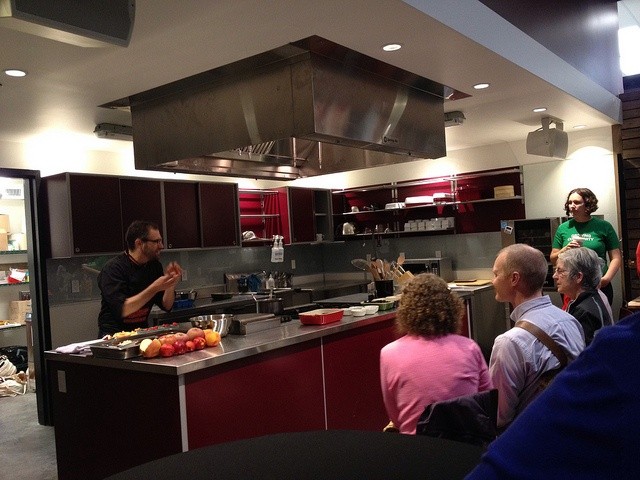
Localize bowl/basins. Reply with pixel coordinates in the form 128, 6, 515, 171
364, 306, 379, 315
175, 291, 197, 303
298, 308, 344, 325
350, 307, 365, 316
188, 314, 234, 338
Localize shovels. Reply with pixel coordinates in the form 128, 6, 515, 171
391, 256, 405, 279
369, 261, 381, 280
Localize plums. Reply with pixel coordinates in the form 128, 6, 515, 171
186, 340, 195, 352
203, 329, 211, 334
175, 341, 186, 354
161, 344, 175, 357
193, 337, 205, 350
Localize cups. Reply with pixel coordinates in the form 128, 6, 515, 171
316, 233, 325, 241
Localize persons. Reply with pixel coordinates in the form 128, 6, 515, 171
554, 245, 603, 346
98, 217, 184, 339
380, 273, 497, 434
488, 244, 586, 427
550, 189, 623, 328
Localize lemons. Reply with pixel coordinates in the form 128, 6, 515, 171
205, 332, 221, 347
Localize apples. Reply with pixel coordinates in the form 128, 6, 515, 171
140, 338, 161, 357
159, 334, 176, 345
187, 327, 205, 340
174, 333, 189, 341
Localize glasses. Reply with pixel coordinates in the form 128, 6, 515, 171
556, 268, 571, 274
144, 238, 162, 244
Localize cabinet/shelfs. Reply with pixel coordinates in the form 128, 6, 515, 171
238, 188, 281, 248
0, 251, 30, 286
277, 186, 332, 246
394, 174, 456, 238
40, 171, 242, 260
330, 181, 396, 239
451, 166, 524, 210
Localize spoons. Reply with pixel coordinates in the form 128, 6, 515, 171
391, 255, 405, 278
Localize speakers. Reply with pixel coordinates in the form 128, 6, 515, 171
525, 117, 569, 160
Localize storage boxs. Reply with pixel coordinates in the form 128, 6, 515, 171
0, 234, 8, 251
8, 300, 32, 323
0, 214, 11, 232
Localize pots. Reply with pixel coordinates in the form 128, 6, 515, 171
274, 272, 293, 288
252, 293, 285, 316
